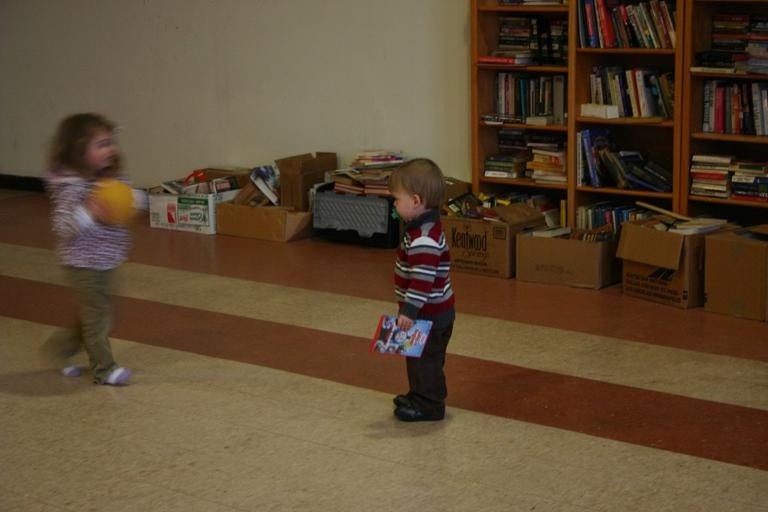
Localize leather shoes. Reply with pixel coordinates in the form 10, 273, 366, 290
393, 391, 419, 408
394, 405, 445, 422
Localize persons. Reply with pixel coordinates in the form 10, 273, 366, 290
388, 158, 455, 422
42, 114, 147, 386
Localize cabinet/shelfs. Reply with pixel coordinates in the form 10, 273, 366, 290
470, 0, 684, 229
683, 0, 768, 227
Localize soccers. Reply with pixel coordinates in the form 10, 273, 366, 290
90, 179, 135, 225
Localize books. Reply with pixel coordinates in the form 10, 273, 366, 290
331, 150, 405, 196
577, 2, 677, 192
686, 1, 768, 201
161, 163, 280, 207
370, 314, 433, 359
447, 190, 572, 239
476, 1, 570, 184
576, 201, 727, 236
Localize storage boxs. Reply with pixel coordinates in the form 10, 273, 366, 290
274, 151, 338, 211
398, 203, 545, 279
215, 199, 313, 242
617, 219, 739, 309
516, 225, 620, 291
705, 225, 768, 321
148, 188, 242, 235
313, 183, 399, 249
